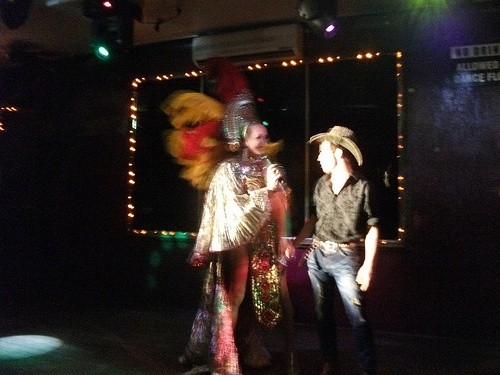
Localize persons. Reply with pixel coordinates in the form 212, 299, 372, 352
189, 122, 290, 375
286, 126, 380, 375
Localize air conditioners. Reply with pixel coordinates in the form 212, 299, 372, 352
192, 23, 304, 69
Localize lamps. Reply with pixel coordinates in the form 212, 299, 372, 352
88, 15, 125, 62
295, 0, 340, 39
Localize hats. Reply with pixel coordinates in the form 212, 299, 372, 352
310, 125, 363, 166
